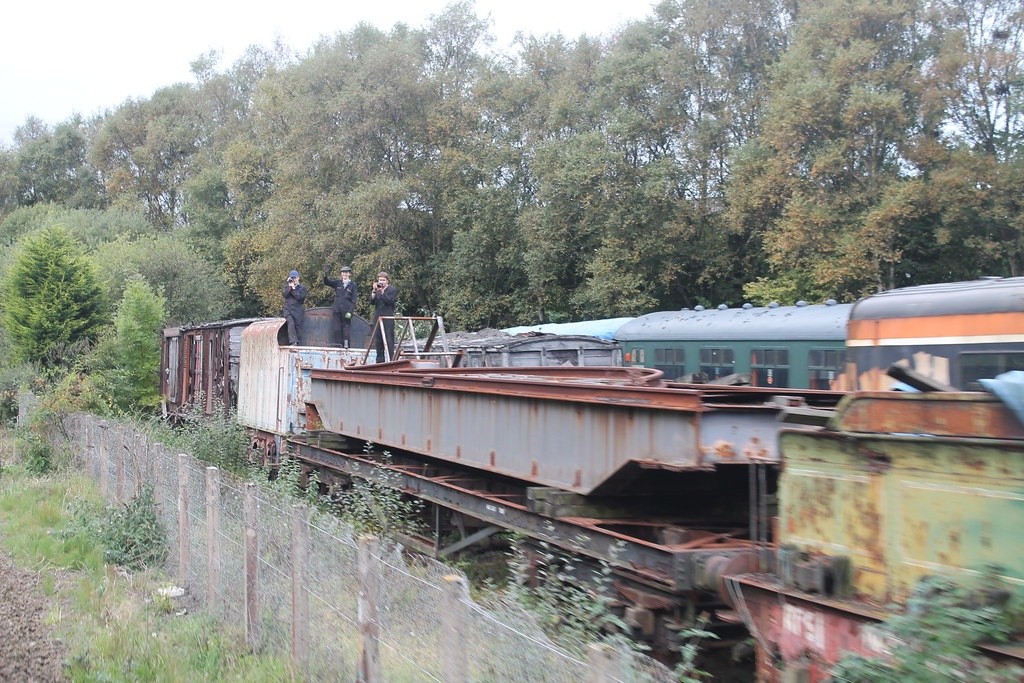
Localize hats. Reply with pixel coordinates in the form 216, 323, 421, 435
377, 272, 389, 280
341, 266, 351, 272
290, 270, 298, 278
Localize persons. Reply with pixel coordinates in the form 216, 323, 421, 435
282, 270, 307, 346
369, 271, 397, 363
323, 266, 358, 347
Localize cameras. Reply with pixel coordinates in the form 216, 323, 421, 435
376, 281, 383, 288
289, 278, 293, 283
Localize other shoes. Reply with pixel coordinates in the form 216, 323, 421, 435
289, 342, 298, 353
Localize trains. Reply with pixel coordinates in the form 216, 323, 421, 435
401, 272, 1024, 395
159, 309, 1024, 683
